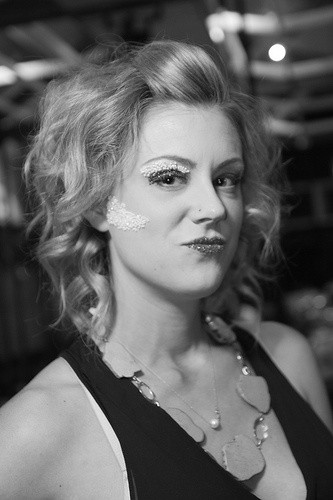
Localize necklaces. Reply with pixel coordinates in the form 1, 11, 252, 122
105, 322, 221, 429
89, 306, 273, 482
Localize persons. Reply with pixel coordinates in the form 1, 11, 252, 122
1, 39, 333, 500
